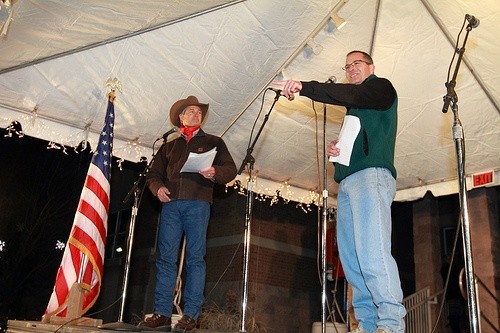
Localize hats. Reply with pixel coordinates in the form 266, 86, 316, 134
170, 96, 209, 128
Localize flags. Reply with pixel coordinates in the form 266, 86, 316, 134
43, 93, 117, 317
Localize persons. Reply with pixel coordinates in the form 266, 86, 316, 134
141, 97, 237, 333
271, 51, 407, 333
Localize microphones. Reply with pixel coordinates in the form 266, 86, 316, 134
156, 127, 178, 141
269, 88, 294, 101
325, 76, 336, 85
464, 14, 480, 28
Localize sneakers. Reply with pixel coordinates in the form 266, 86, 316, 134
175, 315, 200, 330
138, 314, 171, 330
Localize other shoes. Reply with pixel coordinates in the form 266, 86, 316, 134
374, 330, 385, 333
348, 326, 364, 333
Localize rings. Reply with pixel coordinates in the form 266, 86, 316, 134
208, 174, 210, 177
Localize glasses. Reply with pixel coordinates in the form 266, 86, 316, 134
342, 60, 371, 71
